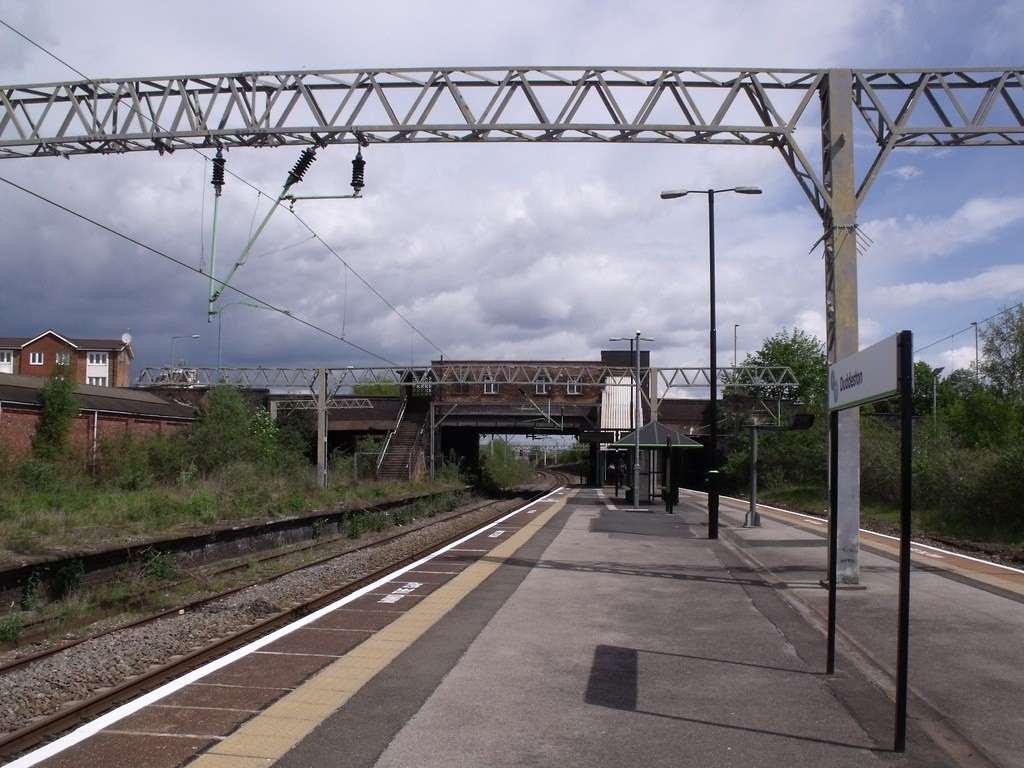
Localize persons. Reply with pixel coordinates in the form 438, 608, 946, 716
618, 458, 627, 489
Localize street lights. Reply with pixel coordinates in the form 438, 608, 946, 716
610, 336, 656, 509
735, 323, 740, 366
170, 334, 200, 381
659, 185, 764, 540
